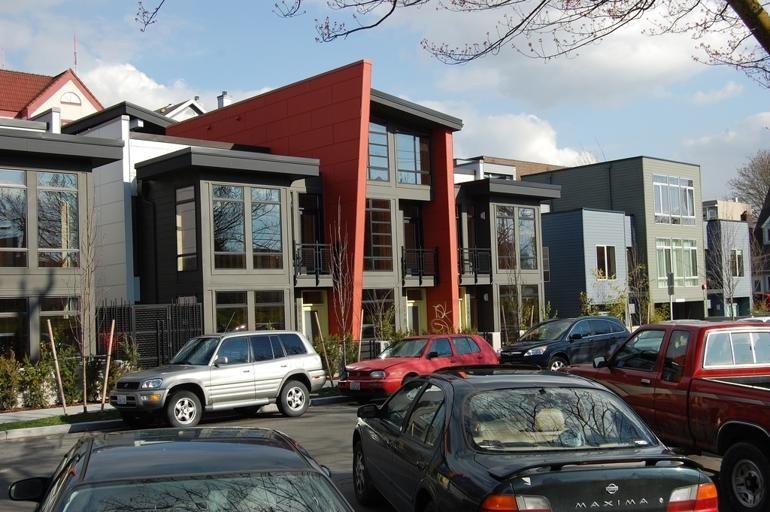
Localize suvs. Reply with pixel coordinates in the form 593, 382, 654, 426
110, 331, 324, 426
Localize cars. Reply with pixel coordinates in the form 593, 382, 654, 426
499, 316, 633, 374
354, 374, 720, 512
337, 334, 497, 405
7, 426, 354, 512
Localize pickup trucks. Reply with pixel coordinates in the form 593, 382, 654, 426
558, 318, 770, 511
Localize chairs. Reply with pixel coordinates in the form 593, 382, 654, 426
533, 402, 565, 432
